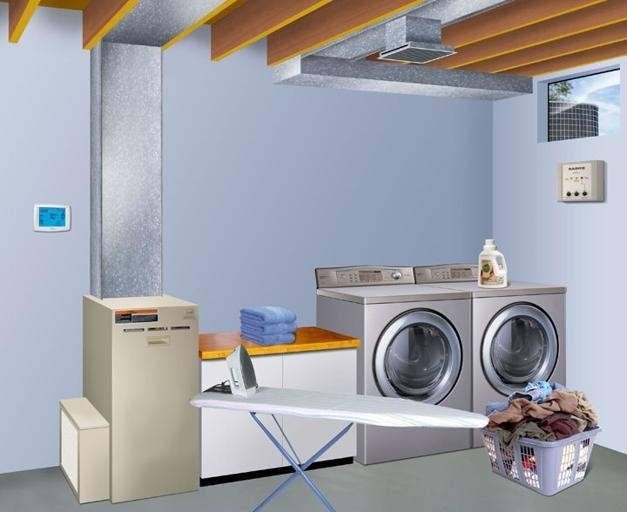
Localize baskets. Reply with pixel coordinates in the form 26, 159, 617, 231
478, 425, 603, 496
